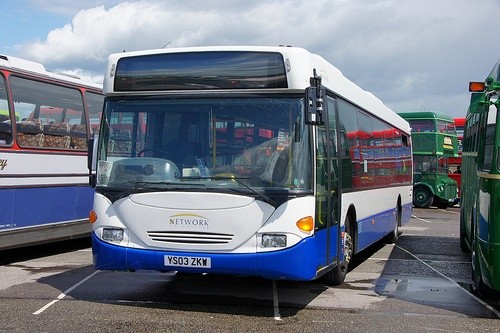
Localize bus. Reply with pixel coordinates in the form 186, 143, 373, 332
88, 45, 415, 287
395, 113, 459, 210
438, 118, 466, 207
0, 53, 105, 254
458, 62, 500, 302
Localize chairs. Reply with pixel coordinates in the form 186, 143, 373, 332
0, 114, 133, 158
482, 124, 500, 168
160, 138, 253, 176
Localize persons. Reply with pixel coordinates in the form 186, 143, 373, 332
453, 166, 462, 174
